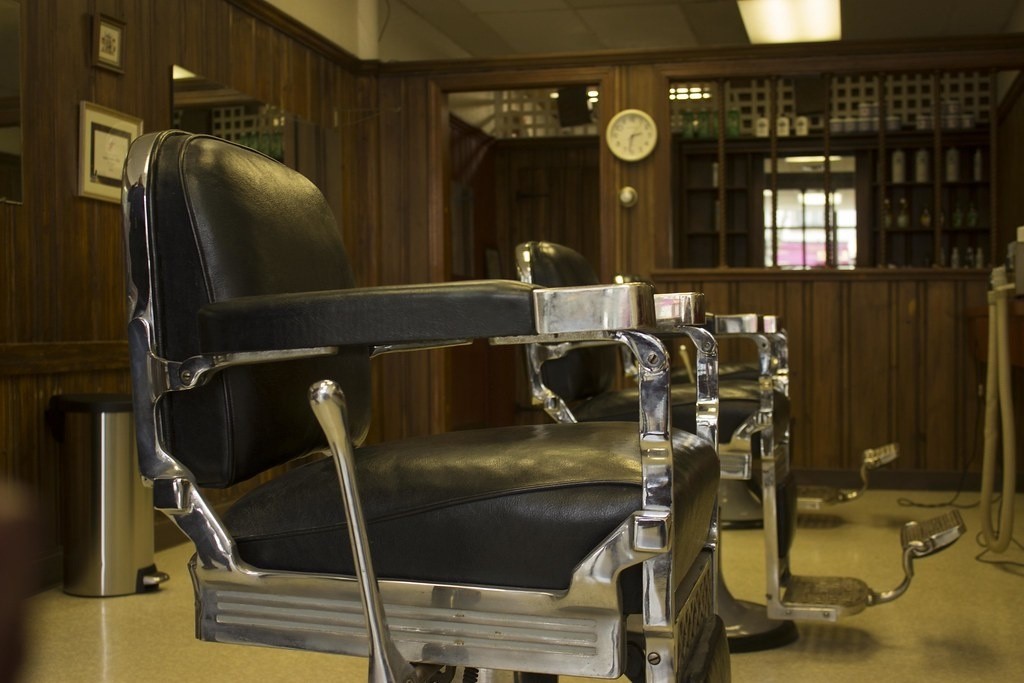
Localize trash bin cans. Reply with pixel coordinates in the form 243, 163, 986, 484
54, 390, 171, 599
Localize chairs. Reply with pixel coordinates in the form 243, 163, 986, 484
513, 239, 968, 622
118, 130, 734, 683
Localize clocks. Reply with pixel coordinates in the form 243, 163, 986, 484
604, 108, 659, 162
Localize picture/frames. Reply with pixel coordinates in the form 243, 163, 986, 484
89, 11, 128, 74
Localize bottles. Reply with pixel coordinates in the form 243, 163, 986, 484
682, 106, 809, 138
964, 247, 974, 267
919, 198, 932, 228
898, 198, 911, 228
950, 248, 960, 269
914, 148, 930, 182
891, 150, 905, 183
945, 148, 961, 181
974, 247, 983, 267
972, 149, 983, 183
952, 197, 964, 228
883, 200, 895, 230
967, 196, 978, 228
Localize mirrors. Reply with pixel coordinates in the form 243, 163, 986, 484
170, 62, 284, 165
0, 0, 24, 208
